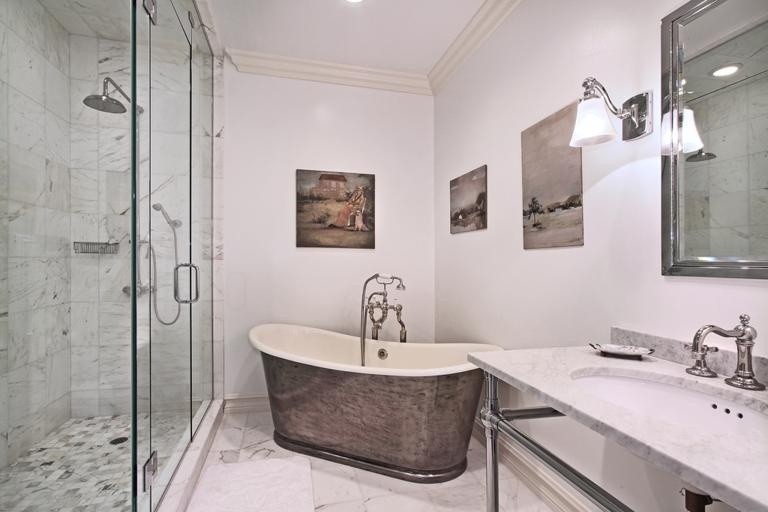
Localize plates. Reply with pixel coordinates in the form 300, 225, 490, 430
599, 344, 654, 359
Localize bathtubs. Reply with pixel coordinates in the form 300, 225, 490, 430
248, 323, 506, 485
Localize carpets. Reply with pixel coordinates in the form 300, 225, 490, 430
187, 455, 316, 512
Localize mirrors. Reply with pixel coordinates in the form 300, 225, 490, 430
659, 0, 768, 278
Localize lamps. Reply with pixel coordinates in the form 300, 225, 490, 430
568, 76, 654, 149
663, 85, 717, 164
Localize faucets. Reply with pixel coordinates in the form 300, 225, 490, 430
688, 312, 765, 390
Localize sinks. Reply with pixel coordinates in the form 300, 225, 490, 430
571, 367, 768, 447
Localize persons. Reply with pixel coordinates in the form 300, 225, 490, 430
328, 185, 364, 228
